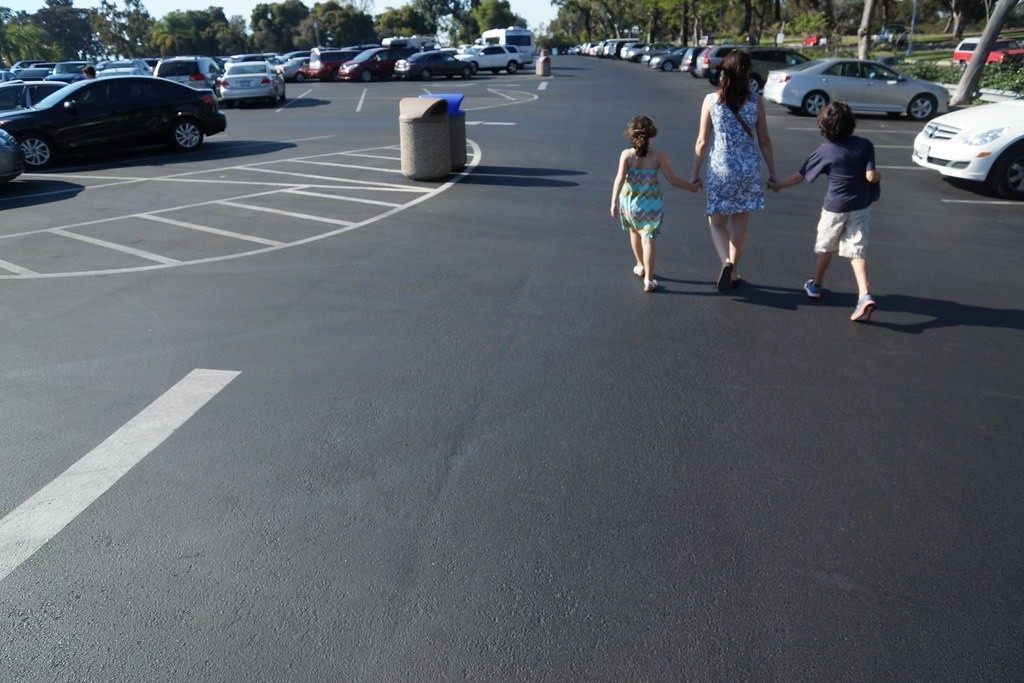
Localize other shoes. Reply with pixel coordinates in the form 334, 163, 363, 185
633, 265, 644, 276
717, 262, 741, 290
644, 279, 659, 292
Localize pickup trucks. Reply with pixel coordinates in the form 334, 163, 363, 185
953, 37, 1024, 68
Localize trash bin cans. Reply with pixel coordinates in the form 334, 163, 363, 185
419, 94, 466, 173
538, 56, 551, 76
398, 98, 451, 180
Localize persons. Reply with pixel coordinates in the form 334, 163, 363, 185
611, 115, 698, 291
82, 66, 97, 101
766, 100, 880, 323
691, 48, 778, 293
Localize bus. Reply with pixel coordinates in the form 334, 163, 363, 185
481, 26, 533, 69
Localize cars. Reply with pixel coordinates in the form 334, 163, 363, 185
273, 37, 523, 80
763, 57, 950, 121
911, 96, 1024, 200
882, 25, 907, 43
701, 44, 762, 86
750, 48, 838, 94
0, 128, 25, 184
567, 38, 716, 78
0, 75, 227, 152
0, 53, 285, 113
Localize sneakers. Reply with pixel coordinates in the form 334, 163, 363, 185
850, 294, 876, 321
804, 278, 823, 297
